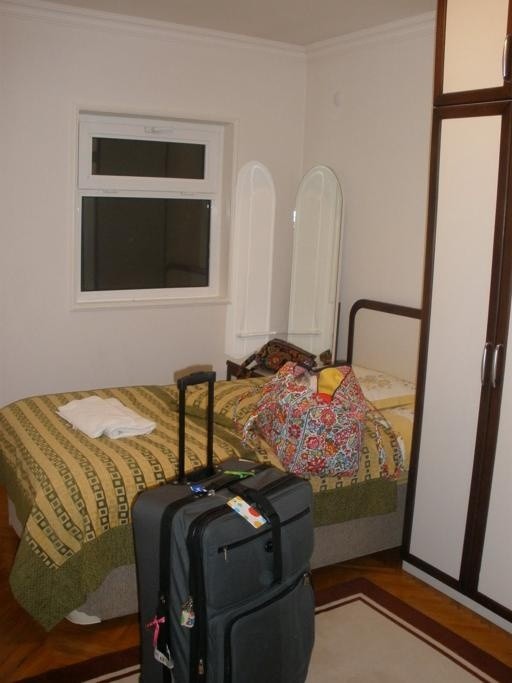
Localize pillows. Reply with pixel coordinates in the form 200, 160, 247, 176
177, 365, 416, 433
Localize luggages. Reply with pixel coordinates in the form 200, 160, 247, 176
130, 457, 316, 682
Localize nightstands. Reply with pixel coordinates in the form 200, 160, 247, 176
225, 339, 285, 380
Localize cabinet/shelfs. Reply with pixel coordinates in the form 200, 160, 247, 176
404, 0, 511, 636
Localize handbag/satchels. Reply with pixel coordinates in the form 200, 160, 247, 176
237, 337, 316, 379
231, 361, 405, 482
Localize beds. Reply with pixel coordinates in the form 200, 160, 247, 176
1, 298, 422, 626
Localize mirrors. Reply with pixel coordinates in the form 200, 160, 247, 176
285, 164, 344, 368
